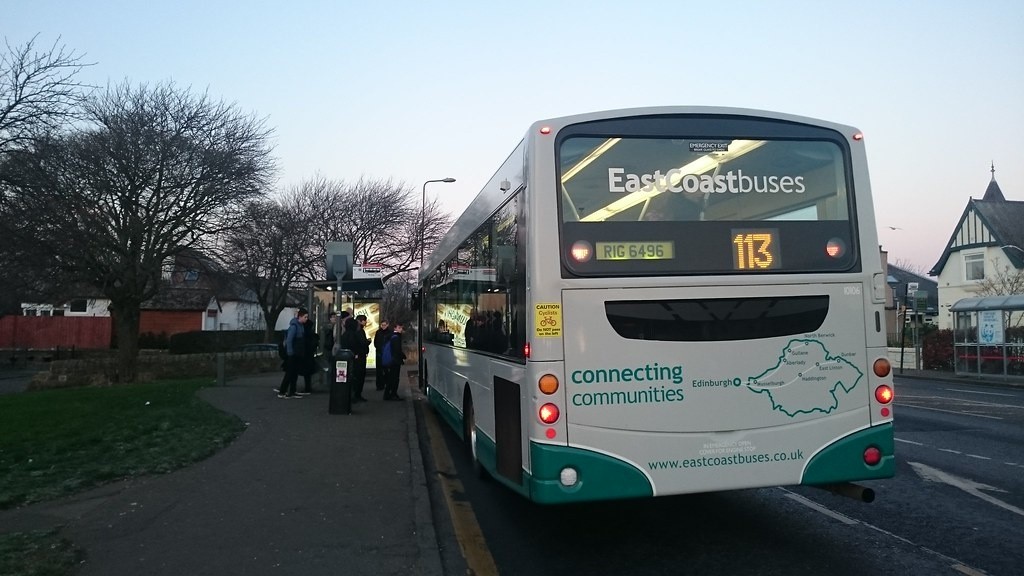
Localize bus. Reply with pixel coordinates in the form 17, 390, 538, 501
410, 105, 900, 509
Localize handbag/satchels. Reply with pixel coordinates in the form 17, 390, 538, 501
278, 324, 298, 359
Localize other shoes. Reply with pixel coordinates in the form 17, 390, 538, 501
354, 397, 367, 402
390, 392, 402, 401
376, 387, 381, 390
273, 388, 310, 398
383, 390, 391, 400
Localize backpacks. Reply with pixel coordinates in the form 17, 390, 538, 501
382, 335, 401, 367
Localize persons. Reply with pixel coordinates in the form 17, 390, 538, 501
430, 319, 455, 347
382, 321, 407, 401
271, 308, 321, 400
324, 309, 372, 404
374, 320, 393, 392
462, 307, 509, 355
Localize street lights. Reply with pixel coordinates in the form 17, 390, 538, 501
914, 291, 929, 372
421, 177, 456, 264
